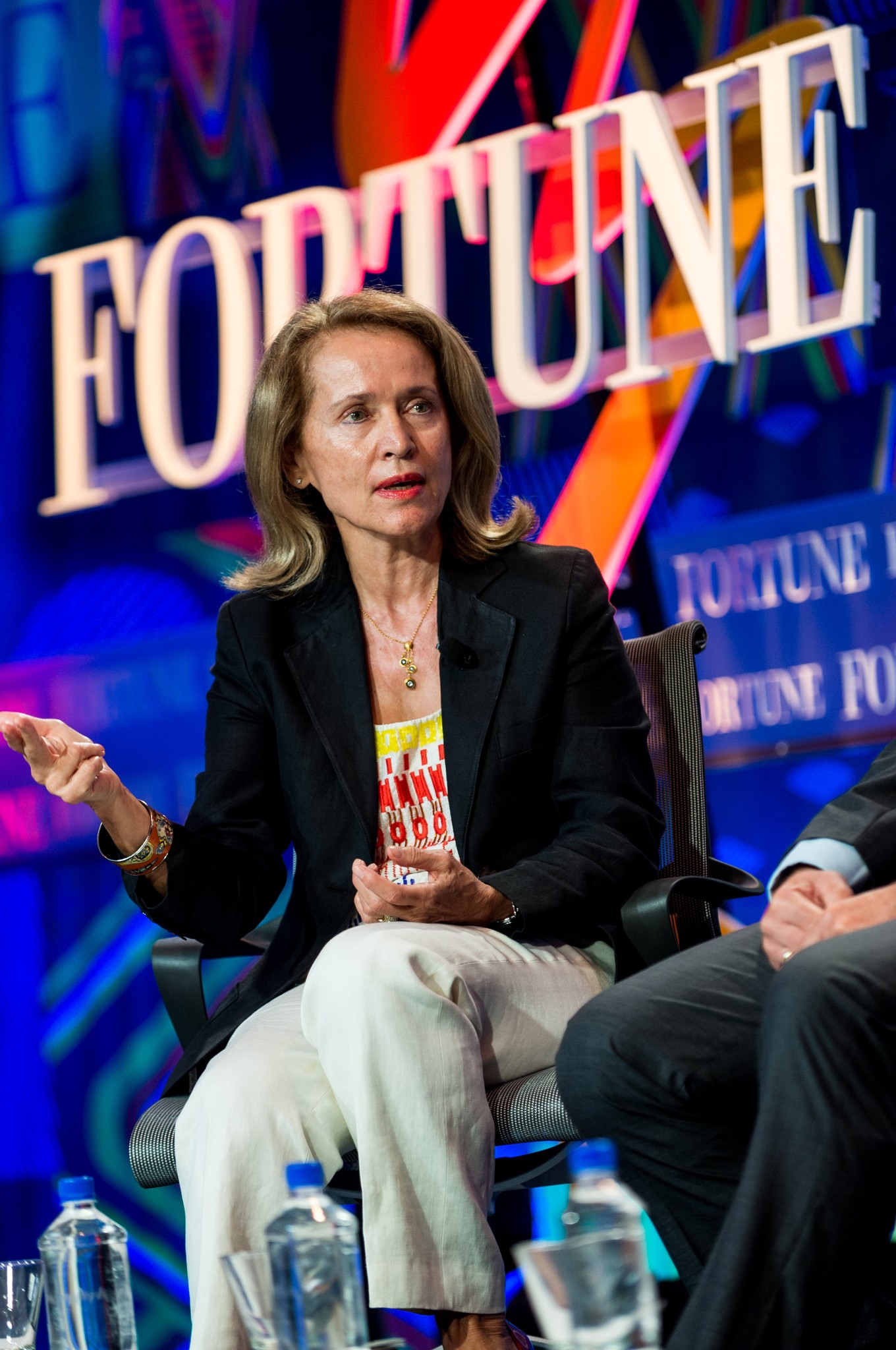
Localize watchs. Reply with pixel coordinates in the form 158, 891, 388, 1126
497, 900, 520, 927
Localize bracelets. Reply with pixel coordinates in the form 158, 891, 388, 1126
96, 798, 174, 877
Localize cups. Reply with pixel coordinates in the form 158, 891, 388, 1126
0, 1259, 45, 1350
224, 1247, 283, 1349
514, 1226, 658, 1350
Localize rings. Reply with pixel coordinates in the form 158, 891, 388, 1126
778, 950, 794, 969
378, 915, 397, 923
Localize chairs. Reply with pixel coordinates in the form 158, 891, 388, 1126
117, 628, 761, 1328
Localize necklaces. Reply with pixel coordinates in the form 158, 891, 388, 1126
355, 582, 438, 691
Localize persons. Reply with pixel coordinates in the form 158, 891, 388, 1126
555, 741, 896, 1350
3, 286, 663, 1350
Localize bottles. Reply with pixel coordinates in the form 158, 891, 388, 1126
36, 1175, 140, 1350
568, 1140, 660, 1350
264, 1162, 368, 1350
